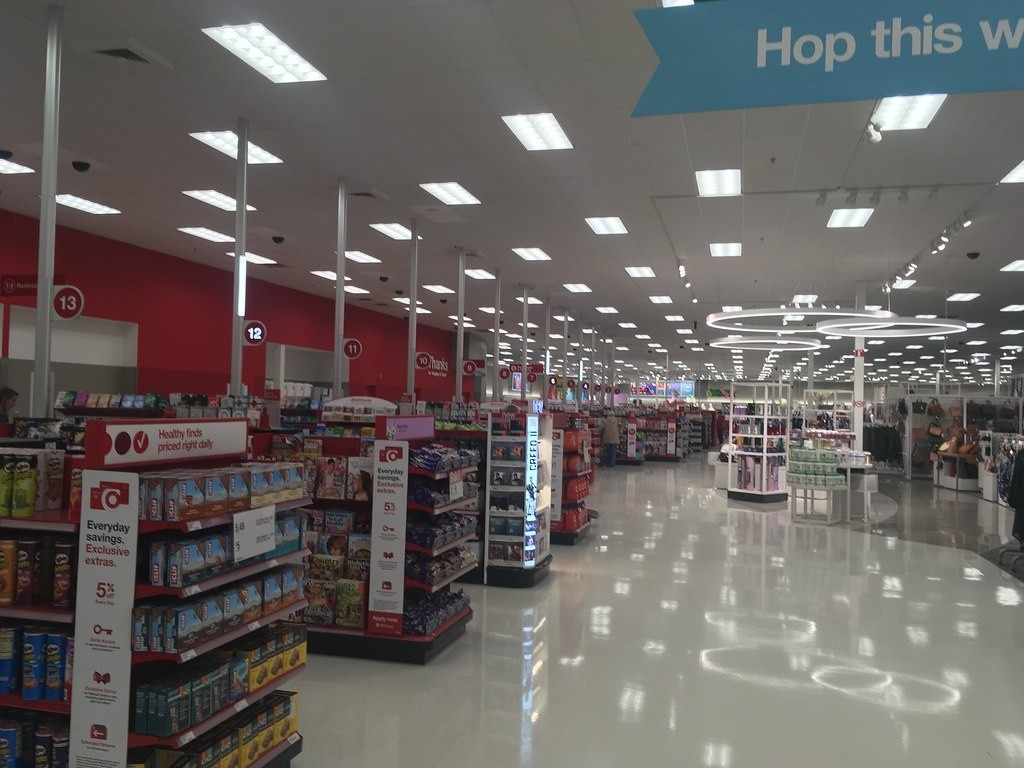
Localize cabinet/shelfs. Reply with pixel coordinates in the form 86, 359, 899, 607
0, 456, 315, 768
977, 430, 1024, 506
281, 409, 591, 665
787, 437, 878, 525
53, 407, 302, 462
934, 452, 978, 491
590, 407, 717, 466
727, 382, 791, 503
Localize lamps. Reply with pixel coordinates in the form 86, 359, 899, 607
896, 188, 909, 204
927, 186, 938, 201
869, 189, 880, 206
815, 192, 827, 206
866, 121, 882, 144
881, 211, 972, 296
845, 190, 857, 206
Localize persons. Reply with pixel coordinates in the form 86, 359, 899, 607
599, 410, 620, 467
270, 447, 372, 627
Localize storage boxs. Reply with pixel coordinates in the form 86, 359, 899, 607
135, 625, 307, 738
149, 513, 301, 588
139, 462, 304, 522
131, 564, 304, 654
127, 690, 299, 768
0, 447, 65, 511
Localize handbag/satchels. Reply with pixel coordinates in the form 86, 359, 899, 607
980, 400, 996, 416
949, 407, 963, 417
999, 400, 1017, 419
912, 427, 926, 441
911, 443, 925, 467
927, 399, 943, 416
927, 419, 1017, 478
912, 398, 927, 414
966, 400, 983, 417
